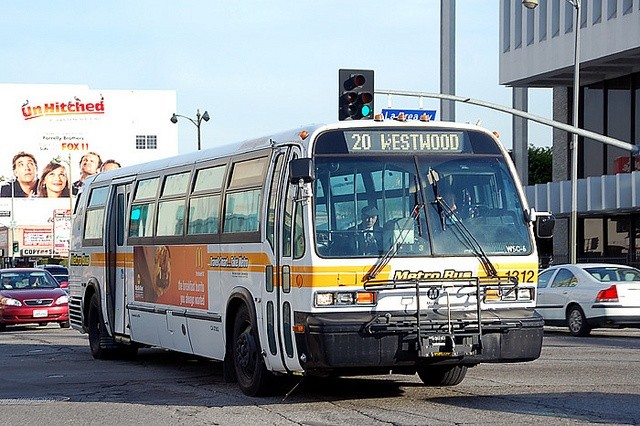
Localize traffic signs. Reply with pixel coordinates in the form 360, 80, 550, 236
381, 108, 436, 122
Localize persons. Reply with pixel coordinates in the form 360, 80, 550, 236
1, 151, 38, 197
100, 158, 121, 172
73, 151, 103, 196
39, 156, 71, 197
346, 205, 384, 255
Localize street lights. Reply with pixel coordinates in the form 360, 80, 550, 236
0, 176, 16, 257
55, 157, 73, 266
522, 1, 582, 265
171, 109, 210, 150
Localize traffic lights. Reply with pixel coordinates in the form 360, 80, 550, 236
13, 241, 18, 253
338, 68, 373, 119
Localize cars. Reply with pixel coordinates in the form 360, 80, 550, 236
36, 264, 72, 293
0, 268, 70, 330
535, 263, 640, 336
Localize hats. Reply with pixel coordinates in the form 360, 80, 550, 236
362, 206, 380, 216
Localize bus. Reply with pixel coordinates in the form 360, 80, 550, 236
68, 116, 556, 400
